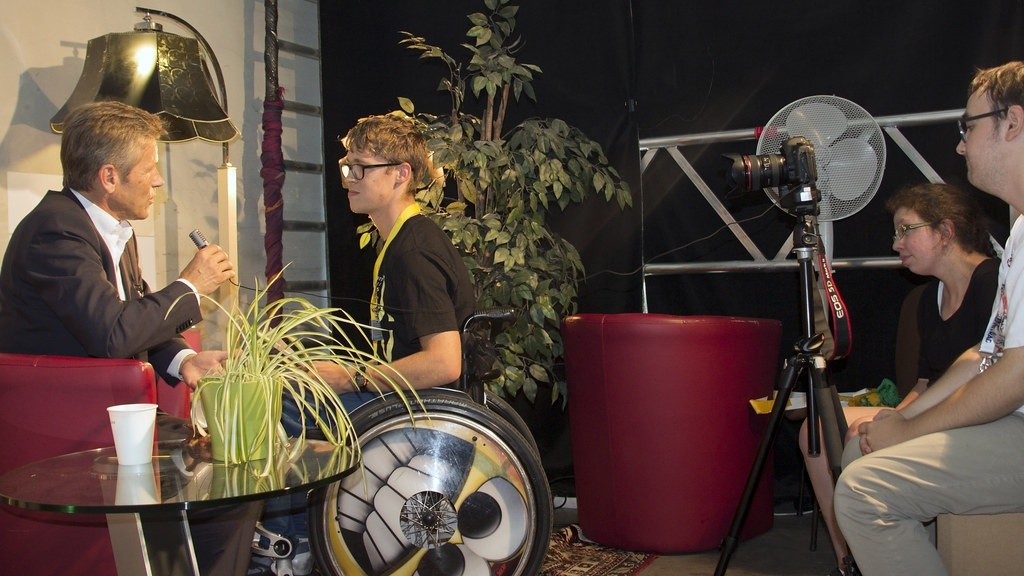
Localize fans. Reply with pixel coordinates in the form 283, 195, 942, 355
755, 95, 887, 326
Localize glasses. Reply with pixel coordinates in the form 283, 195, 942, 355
340, 164, 402, 181
892, 222, 931, 242
957, 108, 1008, 144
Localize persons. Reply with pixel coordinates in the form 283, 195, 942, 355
798, 183, 1002, 576
0, 100, 265, 575
251, 114, 477, 576
833, 62, 1023, 576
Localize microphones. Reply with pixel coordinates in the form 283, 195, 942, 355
188, 228, 209, 249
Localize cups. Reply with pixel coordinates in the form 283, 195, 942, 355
115, 463, 160, 505
107, 403, 158, 465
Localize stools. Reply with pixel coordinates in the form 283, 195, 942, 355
783, 387, 876, 556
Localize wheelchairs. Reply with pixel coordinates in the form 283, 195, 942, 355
250, 308, 554, 576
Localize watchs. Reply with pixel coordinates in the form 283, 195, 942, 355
353, 366, 368, 393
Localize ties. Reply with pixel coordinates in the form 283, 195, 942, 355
117, 259, 151, 364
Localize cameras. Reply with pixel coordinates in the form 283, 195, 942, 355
720, 136, 817, 201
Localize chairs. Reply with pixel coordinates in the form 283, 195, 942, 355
0, 351, 194, 576
560, 313, 784, 556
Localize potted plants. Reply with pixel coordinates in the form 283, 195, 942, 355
161, 263, 432, 464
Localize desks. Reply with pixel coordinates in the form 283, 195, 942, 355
0, 437, 362, 576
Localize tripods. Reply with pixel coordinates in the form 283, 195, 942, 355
713, 200, 850, 576
